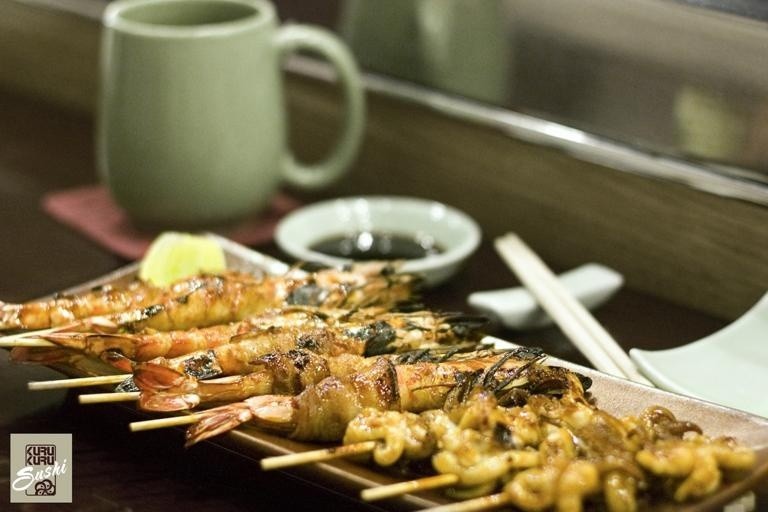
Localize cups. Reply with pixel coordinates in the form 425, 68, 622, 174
95, 2, 365, 236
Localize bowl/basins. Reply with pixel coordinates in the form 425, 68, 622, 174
273, 195, 483, 291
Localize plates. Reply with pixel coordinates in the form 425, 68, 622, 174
43, 189, 302, 260
630, 287, 765, 420
2, 231, 767, 512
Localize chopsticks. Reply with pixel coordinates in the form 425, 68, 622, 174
492, 231, 655, 398
